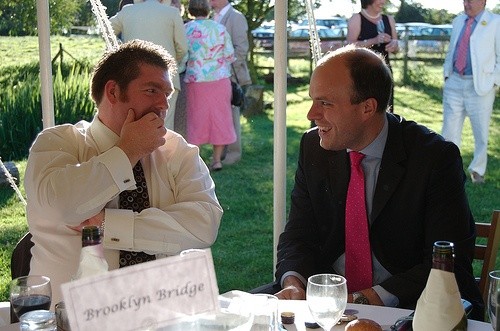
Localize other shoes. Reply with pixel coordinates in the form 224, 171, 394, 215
212, 162, 223, 170
471, 171, 485, 184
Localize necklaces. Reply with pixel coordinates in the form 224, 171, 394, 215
363, 9, 381, 20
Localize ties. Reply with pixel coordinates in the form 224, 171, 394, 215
345, 151, 373, 294
118, 160, 156, 270
454, 18, 474, 76
213, 13, 220, 21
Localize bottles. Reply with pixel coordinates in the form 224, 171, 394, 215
79, 225, 109, 278
413, 241, 468, 331
281, 312, 296, 331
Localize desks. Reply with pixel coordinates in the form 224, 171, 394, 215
0, 300, 492, 331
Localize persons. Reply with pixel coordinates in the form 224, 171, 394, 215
100, 0, 189, 131
246, 44, 476, 308
209, 0, 250, 164
346, 0, 400, 114
440, 0, 500, 183
184, 0, 237, 171
23, 40, 224, 314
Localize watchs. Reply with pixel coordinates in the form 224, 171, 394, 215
352, 290, 369, 305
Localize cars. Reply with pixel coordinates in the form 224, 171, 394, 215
250, 16, 350, 54
396, 22, 454, 53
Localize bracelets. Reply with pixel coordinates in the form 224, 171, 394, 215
99, 218, 105, 237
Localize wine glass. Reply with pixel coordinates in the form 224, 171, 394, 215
306, 273, 348, 331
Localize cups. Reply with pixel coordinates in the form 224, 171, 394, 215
18, 300, 70, 331
10, 274, 52, 320
242, 292, 279, 331
487, 270, 500, 331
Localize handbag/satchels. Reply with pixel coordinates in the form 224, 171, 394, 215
229, 63, 244, 108
